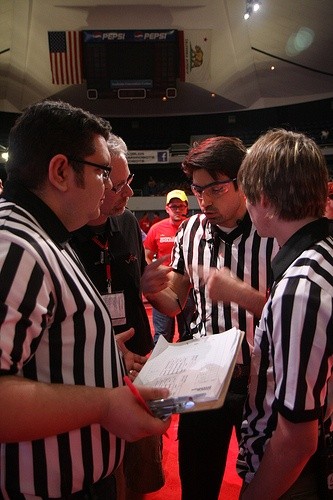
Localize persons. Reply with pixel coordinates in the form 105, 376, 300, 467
151, 212, 161, 226
143, 190, 190, 344
0, 101, 174, 500
139, 211, 151, 235
140, 137, 280, 500
235, 128, 333, 500
69, 132, 166, 499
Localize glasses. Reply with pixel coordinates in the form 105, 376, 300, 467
109, 159, 137, 193
65, 157, 112, 179
190, 177, 237, 199
168, 205, 188, 211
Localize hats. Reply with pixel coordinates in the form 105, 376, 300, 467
166, 189, 189, 204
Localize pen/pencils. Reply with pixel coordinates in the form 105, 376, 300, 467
123, 374, 154, 416
145, 395, 195, 419
